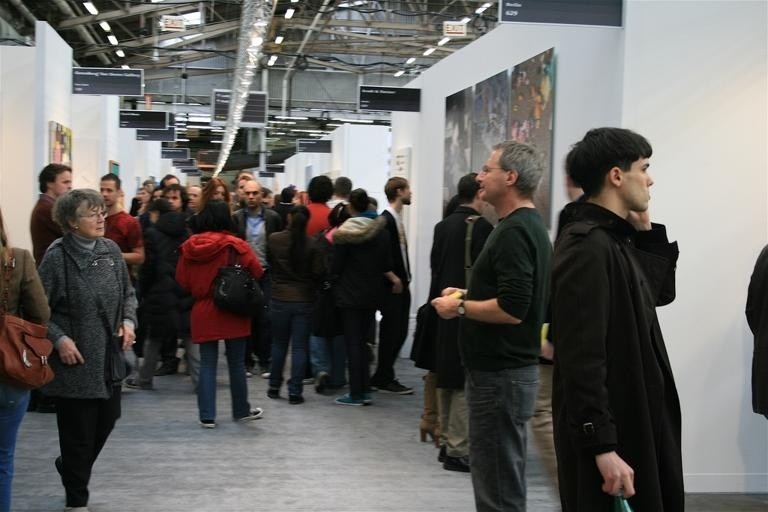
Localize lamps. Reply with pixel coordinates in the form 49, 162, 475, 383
80, 0, 131, 70
265, 1, 304, 67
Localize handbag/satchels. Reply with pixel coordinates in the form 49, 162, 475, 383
105, 336, 128, 383
332, 217, 385, 246
213, 265, 265, 318
331, 266, 382, 311
0, 315, 54, 389
310, 287, 345, 336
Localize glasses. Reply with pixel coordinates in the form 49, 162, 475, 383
79, 210, 108, 220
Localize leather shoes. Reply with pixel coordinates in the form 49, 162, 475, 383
438, 445, 471, 472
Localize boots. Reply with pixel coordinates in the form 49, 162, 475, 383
420, 373, 440, 448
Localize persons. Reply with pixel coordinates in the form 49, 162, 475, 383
431, 141, 554, 512
38, 189, 138, 512
421, 173, 494, 472
1, 212, 49, 512
745, 242, 768, 422
27, 163, 72, 413
554, 124, 687, 512
100, 171, 413, 428
540, 146, 582, 363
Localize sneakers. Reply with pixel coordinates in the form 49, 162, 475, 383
200, 420, 216, 428
261, 371, 415, 406
154, 365, 172, 375
125, 379, 153, 388
240, 407, 263, 420
